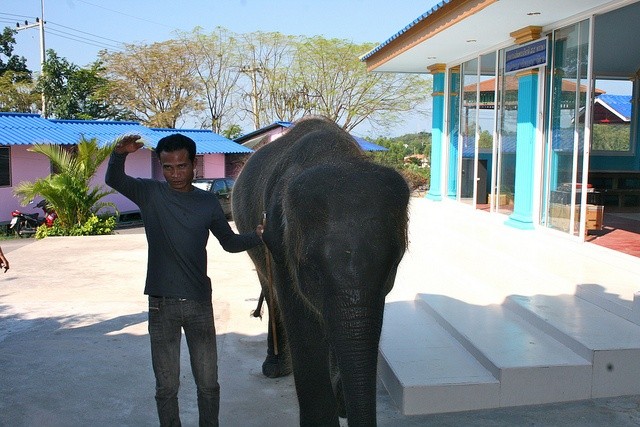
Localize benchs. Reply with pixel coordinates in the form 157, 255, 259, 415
558, 170, 640, 207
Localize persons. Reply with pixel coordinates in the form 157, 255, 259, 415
106, 135, 264, 427
1, 246, 11, 273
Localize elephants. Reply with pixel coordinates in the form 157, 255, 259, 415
231, 117, 410, 427
